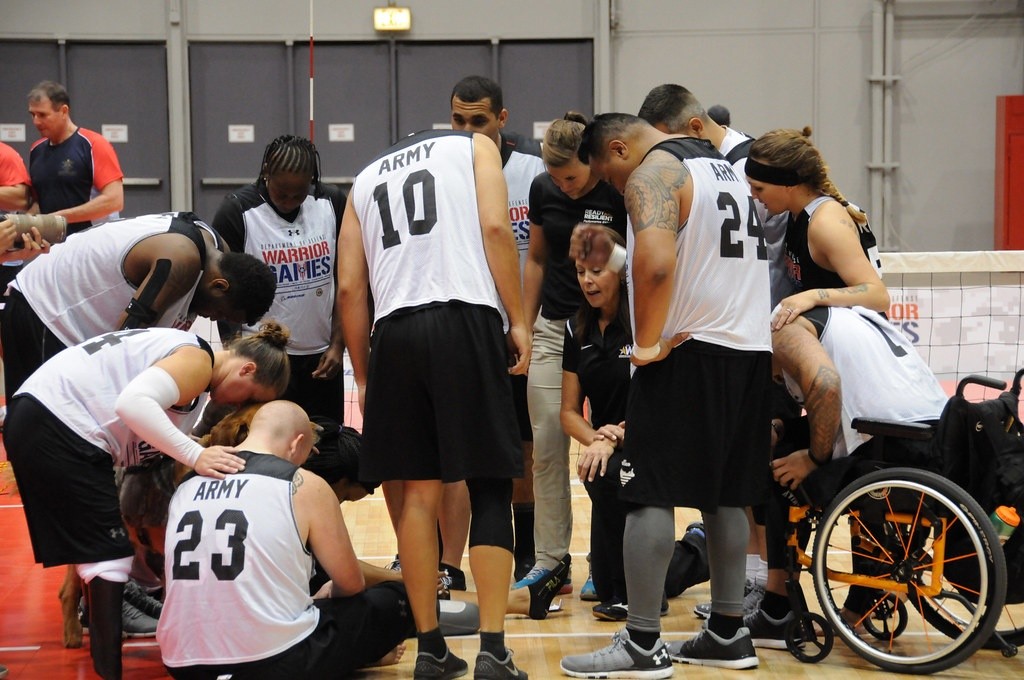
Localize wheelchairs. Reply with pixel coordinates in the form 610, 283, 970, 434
776, 368, 1024, 676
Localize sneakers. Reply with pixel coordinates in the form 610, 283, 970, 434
559, 626, 673, 679
579, 563, 598, 600
413, 643, 468, 680
79, 596, 158, 638
592, 590, 668, 621
701, 600, 805, 649
123, 575, 163, 619
694, 586, 764, 618
473, 647, 529, 680
665, 629, 759, 669
512, 566, 573, 595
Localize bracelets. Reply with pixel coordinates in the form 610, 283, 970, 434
808, 448, 834, 466
632, 339, 662, 361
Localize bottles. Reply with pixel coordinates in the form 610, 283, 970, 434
988, 506, 1020, 547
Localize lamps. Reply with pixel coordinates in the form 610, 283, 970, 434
374, 6, 411, 32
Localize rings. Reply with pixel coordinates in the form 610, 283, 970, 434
786, 308, 792, 315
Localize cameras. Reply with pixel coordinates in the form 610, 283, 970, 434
0, 214, 66, 247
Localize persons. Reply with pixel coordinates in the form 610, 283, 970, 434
559, 111, 776, 680
0, 78, 947, 680
339, 127, 532, 680
